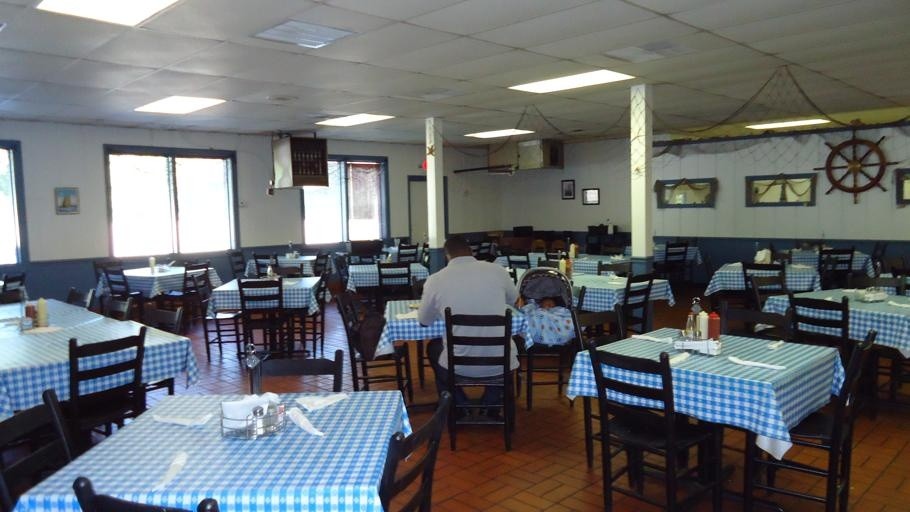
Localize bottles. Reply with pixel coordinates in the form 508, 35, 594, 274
680, 295, 722, 342
265, 241, 300, 279
560, 251, 572, 280
21, 296, 50, 331
148, 255, 157, 274
247, 401, 284, 435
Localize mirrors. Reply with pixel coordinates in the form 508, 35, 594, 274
745, 173, 817, 207
895, 168, 910, 204
656, 178, 715, 208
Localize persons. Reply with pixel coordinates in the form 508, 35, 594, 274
540, 294, 557, 310
411, 235, 526, 431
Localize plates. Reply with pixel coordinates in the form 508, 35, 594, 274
860, 290, 888, 302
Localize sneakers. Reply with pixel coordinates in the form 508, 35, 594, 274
479, 408, 506, 422
458, 408, 474, 422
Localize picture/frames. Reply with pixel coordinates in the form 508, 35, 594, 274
561, 180, 575, 199
582, 188, 600, 205
53, 187, 82, 216
